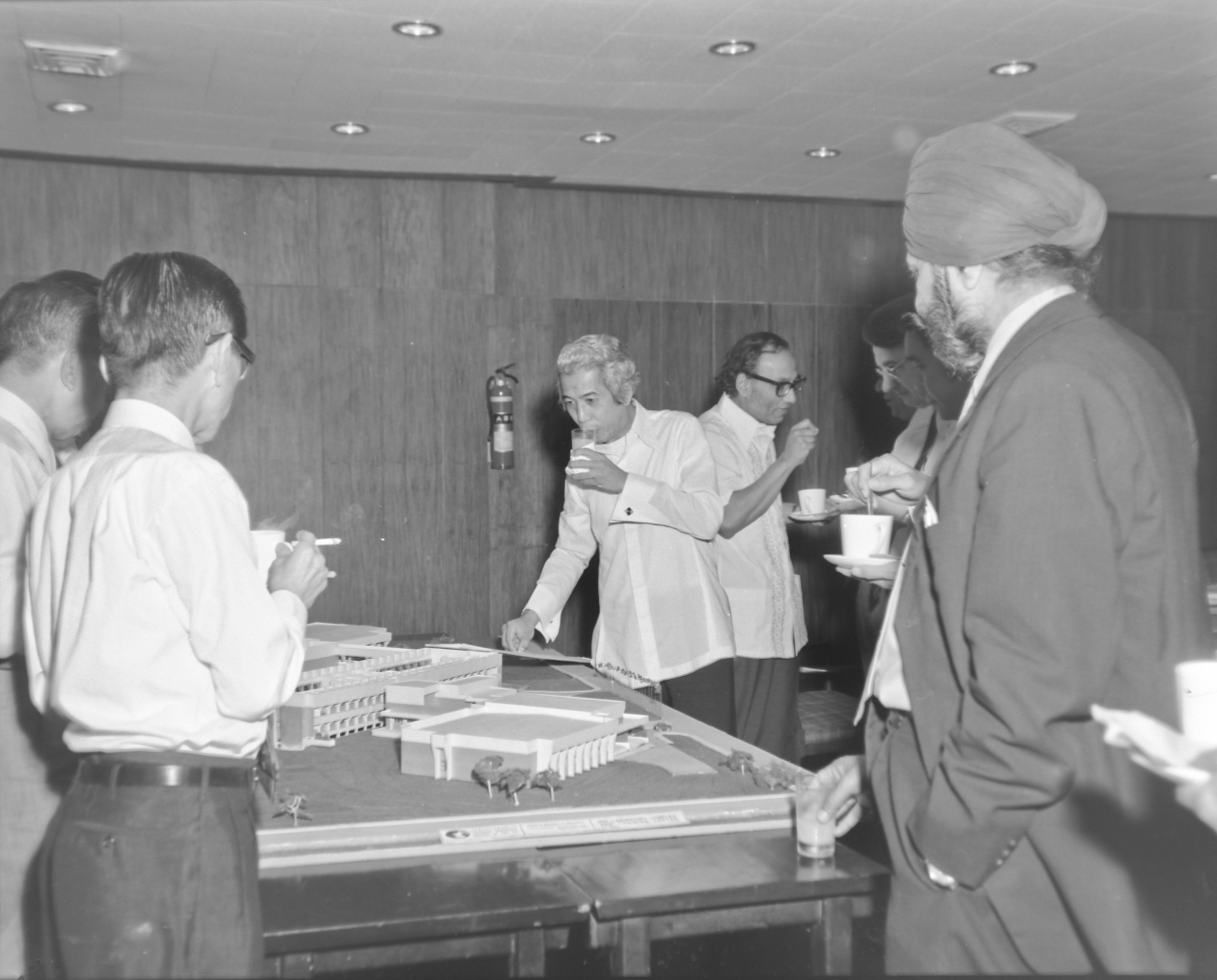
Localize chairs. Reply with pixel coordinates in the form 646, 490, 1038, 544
796, 665, 859, 757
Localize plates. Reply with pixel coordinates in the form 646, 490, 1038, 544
1132, 750, 1210, 783
789, 509, 840, 521
823, 554, 900, 569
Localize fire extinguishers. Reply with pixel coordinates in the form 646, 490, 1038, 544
486, 363, 518, 470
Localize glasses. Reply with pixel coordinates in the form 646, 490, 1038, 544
206, 331, 257, 380
874, 359, 909, 382
744, 369, 806, 397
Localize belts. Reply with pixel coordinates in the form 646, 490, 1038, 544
77, 759, 254, 789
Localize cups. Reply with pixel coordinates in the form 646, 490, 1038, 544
251, 529, 285, 581
1176, 660, 1217, 747
797, 488, 826, 515
571, 427, 596, 473
839, 514, 894, 560
796, 773, 836, 858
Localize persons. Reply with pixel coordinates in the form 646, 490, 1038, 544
841, 291, 971, 675
698, 331, 818, 771
502, 334, 736, 739
853, 122, 1217, 980
22, 252, 329, 980
0, 270, 111, 980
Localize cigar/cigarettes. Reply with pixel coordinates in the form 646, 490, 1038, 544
291, 538, 342, 547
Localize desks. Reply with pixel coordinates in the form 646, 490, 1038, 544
256, 829, 895, 979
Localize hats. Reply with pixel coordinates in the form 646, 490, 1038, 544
902, 123, 1105, 267
